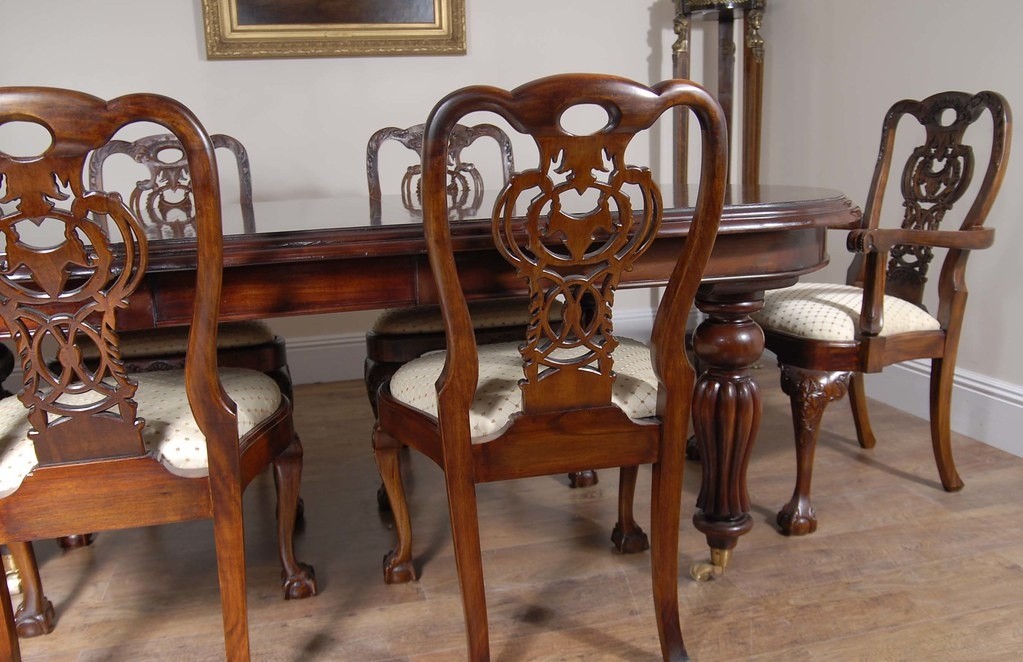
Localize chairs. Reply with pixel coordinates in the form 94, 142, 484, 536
684, 88, 1013, 535
372, 74, 731, 661
1, 86, 317, 662
50, 130, 306, 551
362, 120, 597, 525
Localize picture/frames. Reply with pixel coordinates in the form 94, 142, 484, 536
201, 0, 468, 61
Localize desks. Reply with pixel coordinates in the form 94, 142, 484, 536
1, 179, 863, 595
